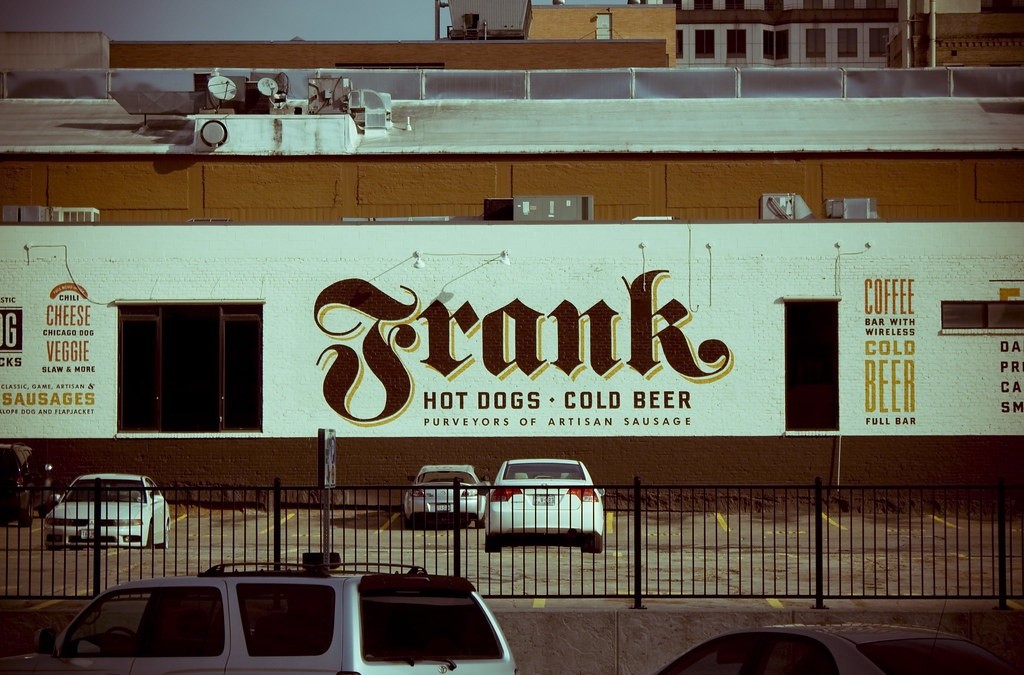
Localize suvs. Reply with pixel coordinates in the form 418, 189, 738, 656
0, 563, 519, 675
0, 442, 64, 527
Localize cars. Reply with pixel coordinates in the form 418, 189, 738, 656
647, 622, 1024, 675
405, 463, 494, 528
487, 460, 607, 554
42, 472, 173, 551
